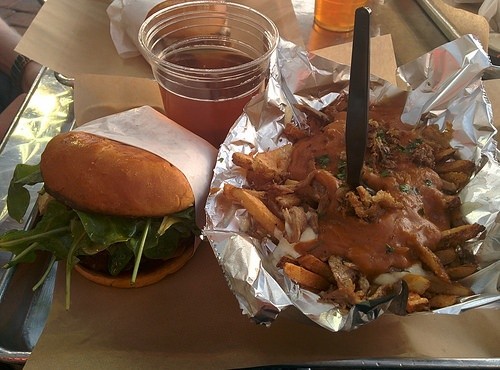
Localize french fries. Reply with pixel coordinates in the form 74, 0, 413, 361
228, 90, 483, 313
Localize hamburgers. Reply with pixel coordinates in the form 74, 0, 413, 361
0, 128, 200, 291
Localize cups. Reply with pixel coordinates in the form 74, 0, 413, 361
314, 0, 371, 32
138, 0, 280, 150
306, 21, 353, 57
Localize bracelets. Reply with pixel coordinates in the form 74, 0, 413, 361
9, 54, 33, 92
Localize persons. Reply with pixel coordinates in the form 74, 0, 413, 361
0, 18, 44, 148
